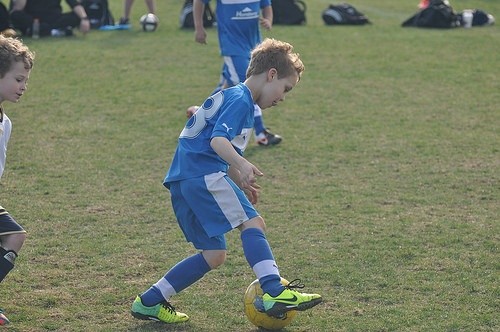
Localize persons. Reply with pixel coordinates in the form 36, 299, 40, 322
130, 39, 322, 323
119, 0, 159, 25
193, 0, 283, 146
0, 35, 34, 324
0, 0, 114, 37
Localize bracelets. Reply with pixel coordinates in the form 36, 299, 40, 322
80, 17, 89, 21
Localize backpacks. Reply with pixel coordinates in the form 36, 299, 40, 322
180, 0, 214, 27
320, 2, 369, 25
262, 0, 307, 24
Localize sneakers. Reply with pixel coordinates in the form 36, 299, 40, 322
130, 294, 190, 324
257, 131, 282, 146
262, 279, 322, 316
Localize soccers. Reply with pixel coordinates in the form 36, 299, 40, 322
1, 27, 17, 38
242, 277, 298, 331
138, 13, 159, 33
